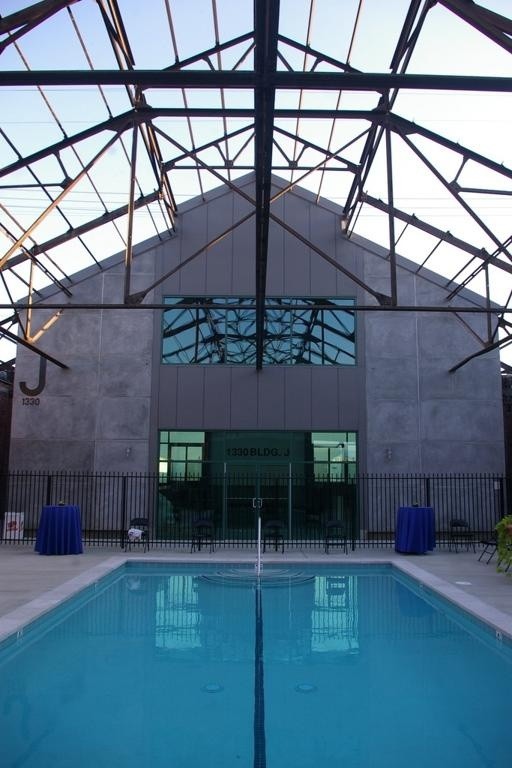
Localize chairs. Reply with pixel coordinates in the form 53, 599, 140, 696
263, 520, 285, 554
189, 518, 215, 553
324, 520, 349, 557
121, 517, 151, 554
447, 519, 476, 554
494, 543, 512, 573
476, 530, 501, 564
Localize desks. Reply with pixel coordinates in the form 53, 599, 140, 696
394, 506, 435, 555
36, 506, 84, 554
